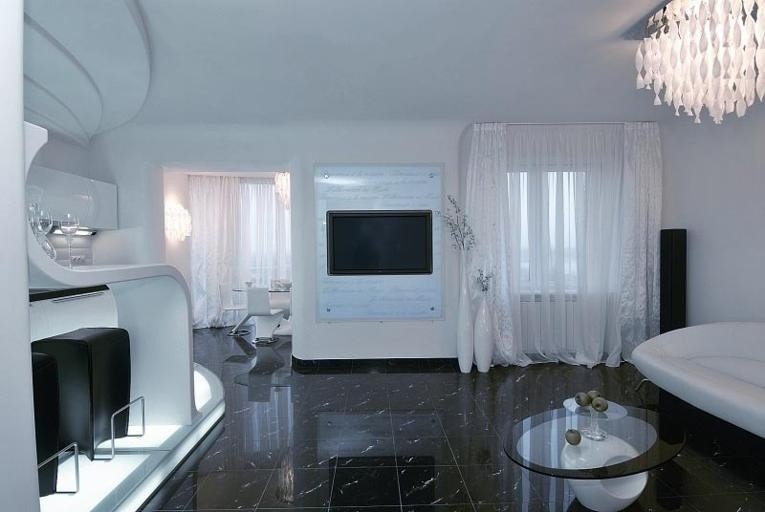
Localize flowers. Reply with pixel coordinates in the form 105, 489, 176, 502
434, 195, 477, 252
476, 267, 495, 292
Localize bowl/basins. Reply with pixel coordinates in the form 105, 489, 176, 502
270, 279, 292, 290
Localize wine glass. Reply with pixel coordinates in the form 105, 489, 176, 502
26, 204, 80, 271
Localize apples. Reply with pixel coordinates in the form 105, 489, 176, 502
576, 406, 608, 419
564, 429, 581, 445
573, 387, 610, 413
566, 446, 582, 459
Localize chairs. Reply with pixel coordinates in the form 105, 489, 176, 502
217, 283, 285, 344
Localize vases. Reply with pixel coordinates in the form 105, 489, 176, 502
457, 251, 474, 373
475, 292, 494, 373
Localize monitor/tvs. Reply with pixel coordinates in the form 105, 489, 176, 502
327, 455, 436, 512
326, 210, 433, 275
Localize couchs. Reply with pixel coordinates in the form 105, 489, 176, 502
632, 321, 765, 482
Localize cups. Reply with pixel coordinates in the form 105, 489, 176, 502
243, 281, 252, 288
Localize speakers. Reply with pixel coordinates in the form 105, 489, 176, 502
657, 388, 684, 445
660, 228, 687, 333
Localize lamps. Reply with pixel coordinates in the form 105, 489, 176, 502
636, 2, 765, 126
